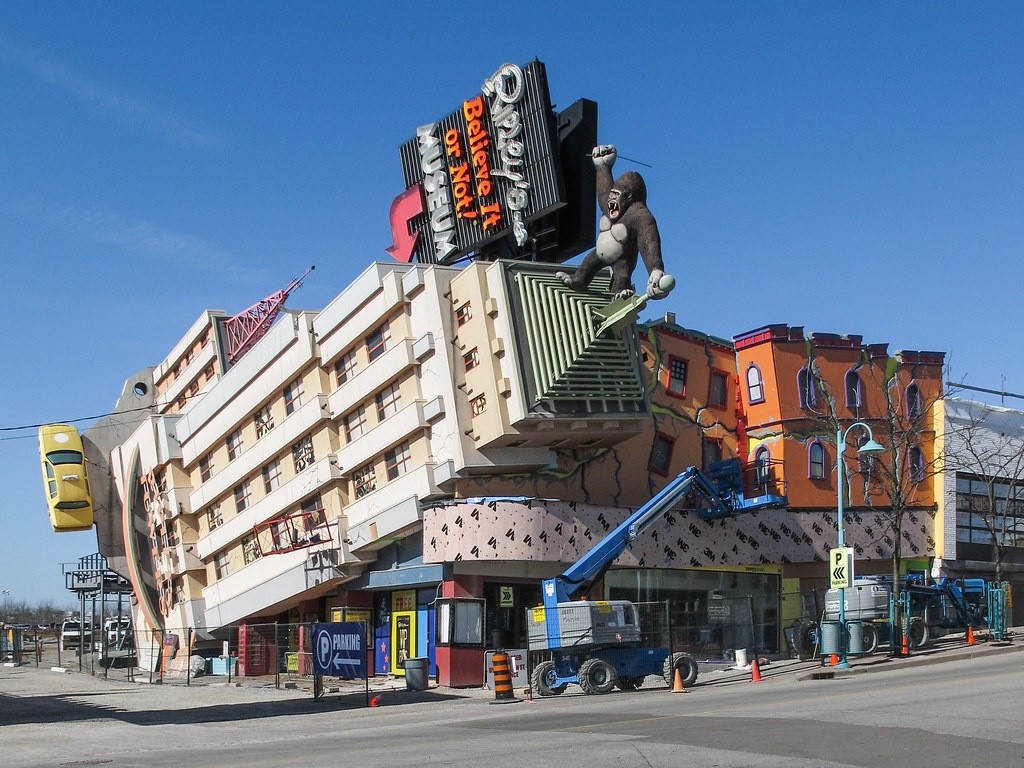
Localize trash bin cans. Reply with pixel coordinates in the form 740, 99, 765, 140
402, 657, 429, 692
820, 619, 842, 655
845, 620, 865, 655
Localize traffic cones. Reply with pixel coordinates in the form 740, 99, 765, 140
830, 655, 838, 665
901, 635, 908, 654
967, 627, 977, 644
751, 659, 764, 682
671, 669, 687, 693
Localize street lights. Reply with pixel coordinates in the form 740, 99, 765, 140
2, 590, 10, 615
836, 423, 886, 669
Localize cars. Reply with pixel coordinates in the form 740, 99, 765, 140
104, 616, 131, 642
38, 423, 94, 533
4, 624, 49, 629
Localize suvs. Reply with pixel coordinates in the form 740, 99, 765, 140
61, 620, 92, 652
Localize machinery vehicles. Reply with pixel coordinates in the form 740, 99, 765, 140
526, 456, 791, 698
825, 568, 989, 655
